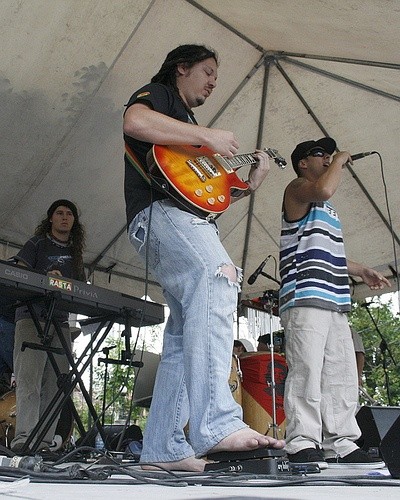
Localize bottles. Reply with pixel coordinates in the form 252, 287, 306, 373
274, 336, 280, 352
95, 432, 105, 449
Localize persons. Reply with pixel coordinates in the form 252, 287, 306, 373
233, 338, 254, 357
278, 136, 393, 469
349, 324, 365, 387
121, 42, 289, 474
12, 198, 89, 462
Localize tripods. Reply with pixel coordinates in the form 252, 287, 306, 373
264, 307, 285, 440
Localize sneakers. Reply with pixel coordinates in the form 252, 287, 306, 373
286, 446, 328, 468
325, 447, 385, 468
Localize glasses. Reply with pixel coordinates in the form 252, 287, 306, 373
306, 149, 326, 157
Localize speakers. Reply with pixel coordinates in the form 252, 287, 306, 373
355, 405, 400, 447
74, 424, 143, 454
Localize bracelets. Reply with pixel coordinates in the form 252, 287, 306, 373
241, 178, 255, 197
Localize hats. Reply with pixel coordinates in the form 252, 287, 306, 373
291, 136, 337, 165
47, 199, 78, 218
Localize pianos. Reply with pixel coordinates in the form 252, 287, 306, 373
0, 258, 165, 466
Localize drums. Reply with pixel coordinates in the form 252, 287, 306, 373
184, 352, 242, 462
238, 351, 288, 438
0, 390, 73, 455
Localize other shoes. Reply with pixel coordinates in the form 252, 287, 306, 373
37, 448, 59, 459
13, 443, 31, 455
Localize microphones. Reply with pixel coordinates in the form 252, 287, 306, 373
343, 152, 374, 165
248, 255, 271, 285
361, 302, 371, 307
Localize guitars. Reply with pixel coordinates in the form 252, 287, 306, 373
145, 143, 287, 218
